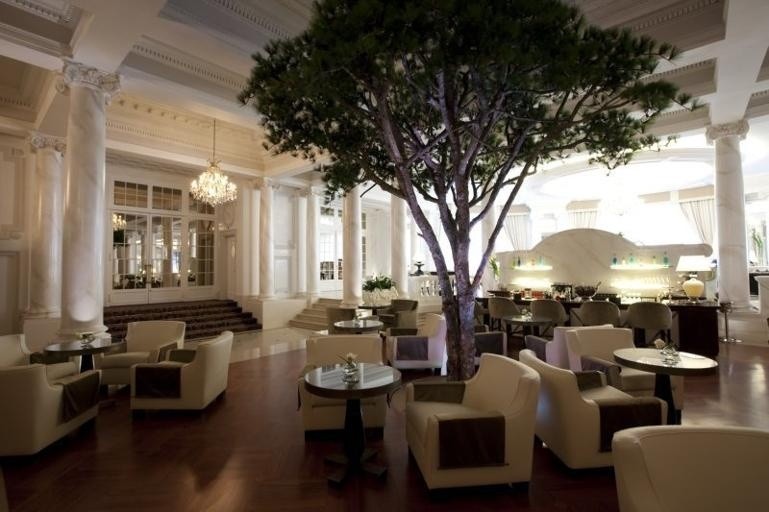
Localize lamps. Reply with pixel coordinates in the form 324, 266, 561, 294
676, 254, 712, 302
187, 117, 239, 207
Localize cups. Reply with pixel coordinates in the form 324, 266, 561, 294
514, 292, 521, 301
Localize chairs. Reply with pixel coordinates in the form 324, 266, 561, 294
475, 297, 674, 349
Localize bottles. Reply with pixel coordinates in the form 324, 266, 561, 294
549, 292, 552, 298
312, 365, 321, 384
544, 291, 548, 298
628, 252, 634, 263
663, 252, 669, 264
359, 363, 369, 384
524, 289, 533, 298
611, 253, 618, 265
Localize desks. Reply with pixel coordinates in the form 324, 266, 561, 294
749, 273, 769, 296
